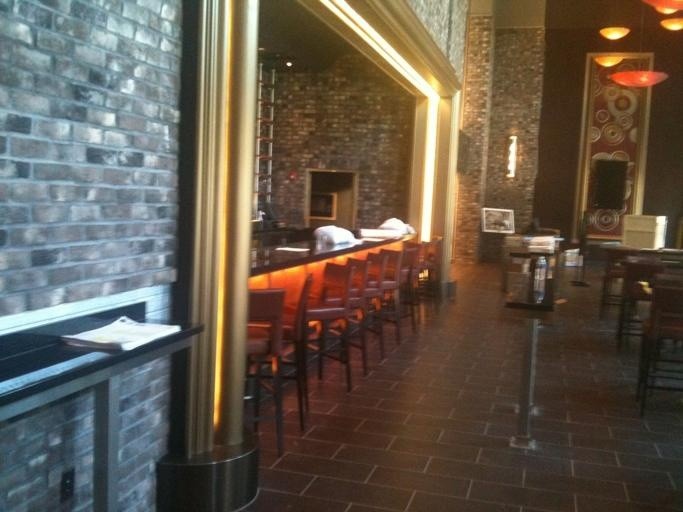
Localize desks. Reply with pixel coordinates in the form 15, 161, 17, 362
0, 317, 205, 512
505, 227, 561, 312
570, 237, 622, 286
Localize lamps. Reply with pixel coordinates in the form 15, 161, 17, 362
503, 135, 517, 179
593, 0, 683, 88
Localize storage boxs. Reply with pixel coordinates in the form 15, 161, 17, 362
622, 214, 667, 250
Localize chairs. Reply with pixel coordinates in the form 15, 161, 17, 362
244, 236, 443, 456
602, 242, 682, 418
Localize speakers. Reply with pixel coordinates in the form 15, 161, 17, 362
592, 160, 627, 209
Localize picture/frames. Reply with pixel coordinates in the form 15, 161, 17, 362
310, 191, 337, 221
